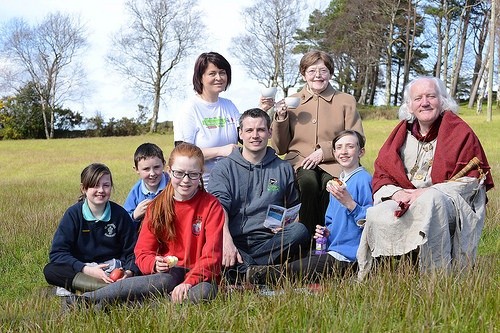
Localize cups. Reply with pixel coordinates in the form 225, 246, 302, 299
261, 86, 277, 99
284, 97, 300, 109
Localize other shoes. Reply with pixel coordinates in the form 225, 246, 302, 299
247, 264, 283, 284
65, 295, 89, 307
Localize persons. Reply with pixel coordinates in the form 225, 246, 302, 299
66, 141, 226, 305
173, 48, 375, 288
42, 162, 143, 291
121, 142, 170, 232
355, 74, 495, 285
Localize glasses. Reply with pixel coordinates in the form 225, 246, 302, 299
307, 68, 330, 76
169, 168, 202, 180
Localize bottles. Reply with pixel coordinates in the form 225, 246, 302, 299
315, 228, 327, 254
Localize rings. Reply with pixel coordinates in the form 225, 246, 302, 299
282, 106, 287, 110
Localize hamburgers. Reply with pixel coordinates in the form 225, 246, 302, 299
326, 178, 346, 191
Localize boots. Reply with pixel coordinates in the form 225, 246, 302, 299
72, 273, 109, 292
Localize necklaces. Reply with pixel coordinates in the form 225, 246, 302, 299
409, 135, 428, 182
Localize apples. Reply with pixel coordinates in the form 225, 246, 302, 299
163, 256, 178, 268
109, 268, 124, 282
